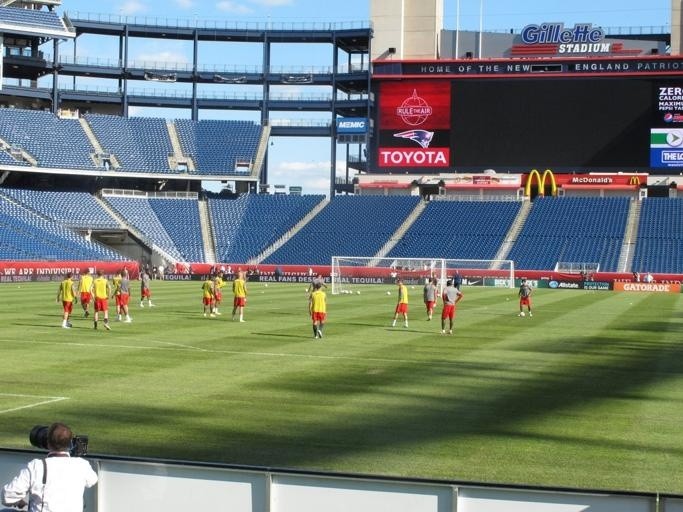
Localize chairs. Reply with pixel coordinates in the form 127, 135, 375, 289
1, 6, 76, 41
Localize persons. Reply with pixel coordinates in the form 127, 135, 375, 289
306, 266, 333, 337
585, 273, 594, 281
633, 271, 640, 282
518, 279, 533, 318
57, 259, 184, 330
438, 281, 463, 334
643, 272, 653, 282
201, 264, 280, 323
430, 272, 438, 308
390, 270, 397, 284
391, 280, 410, 328
2, 422, 98, 512
424, 277, 437, 320
451, 270, 463, 290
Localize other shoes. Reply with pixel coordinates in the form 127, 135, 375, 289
240, 319, 246, 323
450, 330, 452, 335
85, 311, 88, 318
126, 314, 131, 322
210, 314, 215, 317
212, 308, 215, 313
440, 330, 445, 334
427, 315, 431, 320
529, 312, 532, 317
314, 336, 318, 339
392, 322, 395, 327
149, 304, 155, 307
318, 330, 322, 338
405, 325, 408, 329
140, 301, 144, 308
104, 324, 111, 330
118, 314, 122, 321
518, 312, 524, 317
204, 313, 206, 317
63, 322, 71, 329
94, 321, 97, 329
216, 312, 221, 315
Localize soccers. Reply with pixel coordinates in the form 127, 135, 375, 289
265, 283, 269, 287
305, 289, 308, 293
339, 289, 361, 294
386, 291, 391, 296
